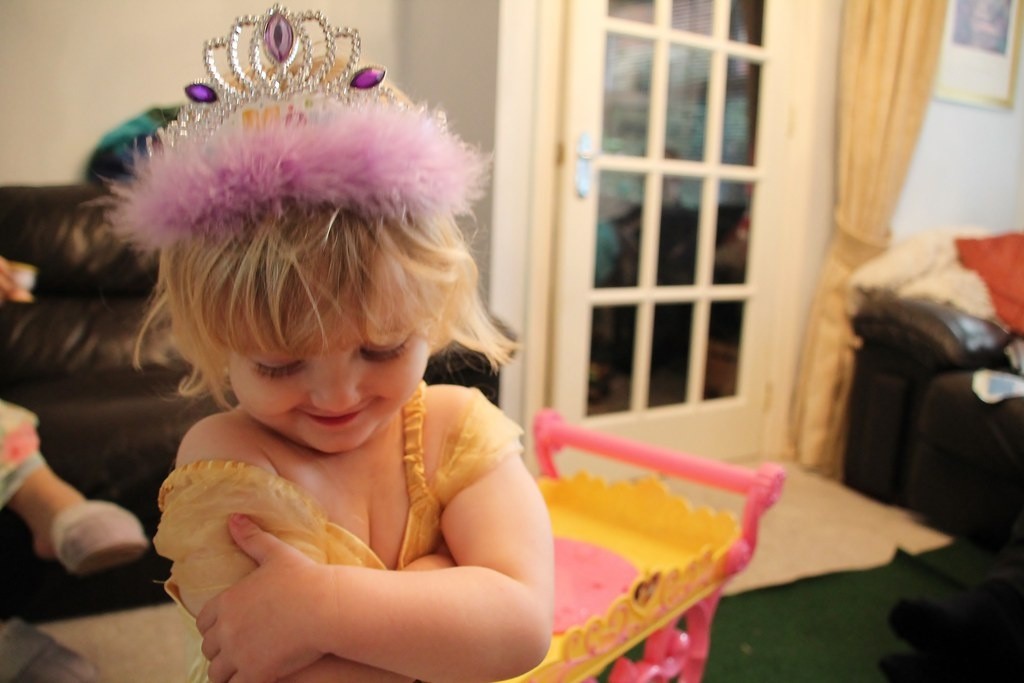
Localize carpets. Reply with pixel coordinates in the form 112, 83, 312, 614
721, 456, 955, 599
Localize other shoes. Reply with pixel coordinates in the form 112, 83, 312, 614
879, 601, 1012, 683
51, 500, 149, 575
0, 617, 100, 683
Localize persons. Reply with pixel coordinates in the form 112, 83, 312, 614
108, 2, 553, 683
0, 257, 149, 574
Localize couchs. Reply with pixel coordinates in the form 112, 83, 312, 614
0, 184, 501, 627
842, 300, 1024, 534
